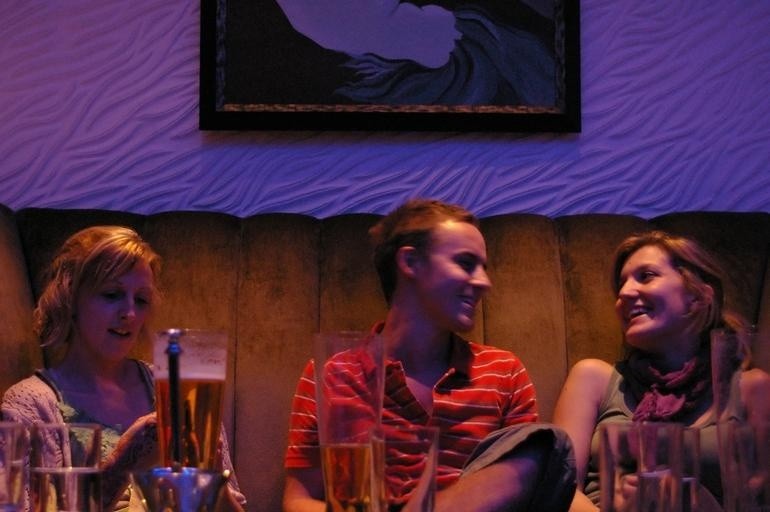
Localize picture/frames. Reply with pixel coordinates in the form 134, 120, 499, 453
198, 0, 582, 135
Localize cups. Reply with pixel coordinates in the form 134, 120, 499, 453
154, 327, 230, 511
312, 328, 385, 512
370, 423, 437, 512
33, 422, 103, 512
640, 421, 682, 512
0, 421, 27, 512
710, 325, 770, 511
680, 428, 702, 512
598, 423, 637, 512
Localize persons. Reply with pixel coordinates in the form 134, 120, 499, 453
0, 226, 245, 512
549, 232, 766, 512
281, 198, 578, 512
